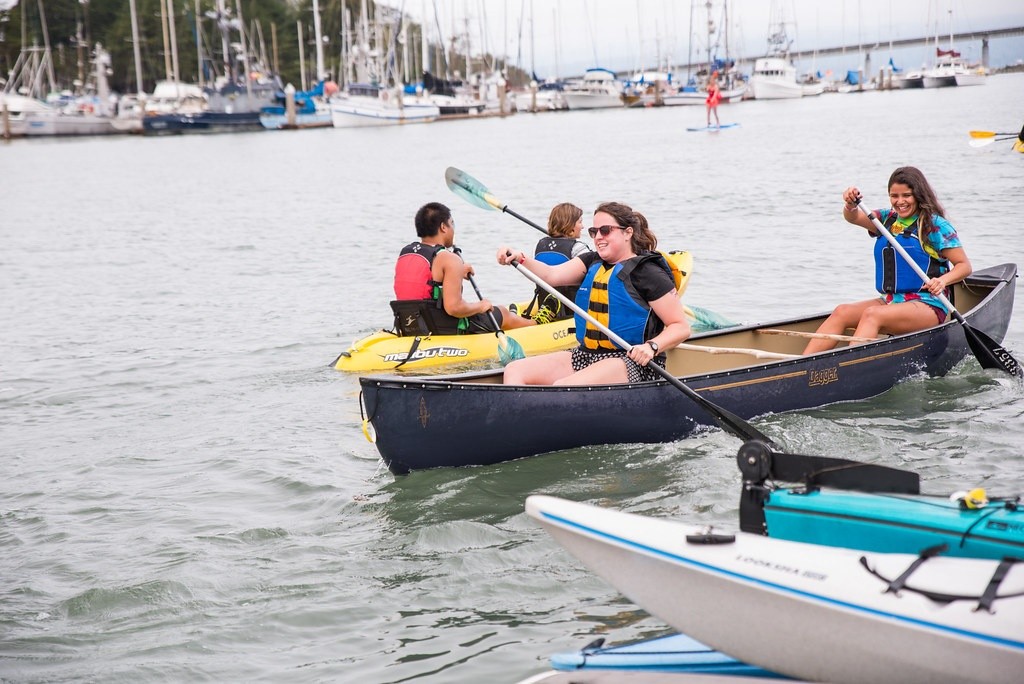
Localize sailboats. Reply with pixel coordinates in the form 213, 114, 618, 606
0, 0, 993, 139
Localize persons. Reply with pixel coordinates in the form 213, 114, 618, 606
536, 203, 593, 260
394, 202, 561, 336
803, 167, 972, 354
496, 201, 690, 387
706, 71, 722, 127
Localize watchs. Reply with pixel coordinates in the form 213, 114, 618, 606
647, 341, 658, 355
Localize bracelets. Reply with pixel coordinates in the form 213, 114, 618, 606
519, 252, 525, 264
845, 204, 858, 212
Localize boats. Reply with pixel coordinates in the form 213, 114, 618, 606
358, 262, 1020, 477
503, 439, 1023, 684
331, 248, 695, 371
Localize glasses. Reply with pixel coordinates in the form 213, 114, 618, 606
588, 224, 625, 239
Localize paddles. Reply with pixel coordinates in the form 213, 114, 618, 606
445, 166, 738, 329
450, 244, 526, 366
854, 191, 1024, 380
505, 250, 784, 454
969, 131, 1022, 147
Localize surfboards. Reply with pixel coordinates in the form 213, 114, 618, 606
685, 123, 738, 131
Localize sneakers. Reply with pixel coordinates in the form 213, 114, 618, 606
531, 294, 561, 325
